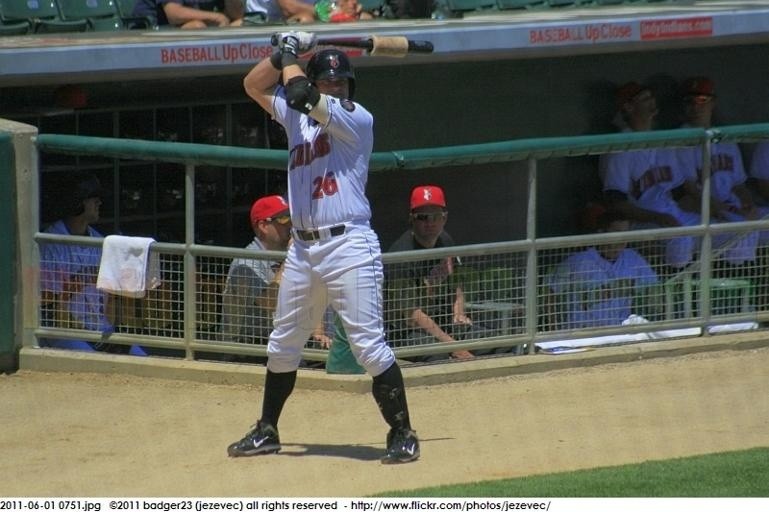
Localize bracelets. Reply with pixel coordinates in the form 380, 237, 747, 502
271, 52, 283, 72
280, 54, 298, 69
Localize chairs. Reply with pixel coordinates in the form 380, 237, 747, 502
0, 1, 696, 37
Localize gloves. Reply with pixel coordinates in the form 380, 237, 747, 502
274, 31, 315, 56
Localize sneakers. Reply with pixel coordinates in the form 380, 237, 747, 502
380, 429, 420, 463
228, 420, 281, 456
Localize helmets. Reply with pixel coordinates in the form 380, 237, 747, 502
305, 50, 355, 79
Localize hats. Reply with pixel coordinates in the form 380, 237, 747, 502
409, 186, 446, 211
250, 196, 289, 227
615, 76, 719, 110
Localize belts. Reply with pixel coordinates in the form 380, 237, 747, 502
292, 225, 348, 242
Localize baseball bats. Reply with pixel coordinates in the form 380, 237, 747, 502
271, 35, 433, 57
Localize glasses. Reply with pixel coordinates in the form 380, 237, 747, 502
412, 212, 444, 221
270, 213, 291, 225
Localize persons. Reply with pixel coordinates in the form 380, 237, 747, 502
600, 83, 741, 281
667, 77, 769, 266
216, 194, 336, 350
540, 212, 665, 339
39, 177, 150, 359
752, 138, 769, 209
382, 184, 508, 363
133, 1, 457, 34
228, 28, 421, 466
